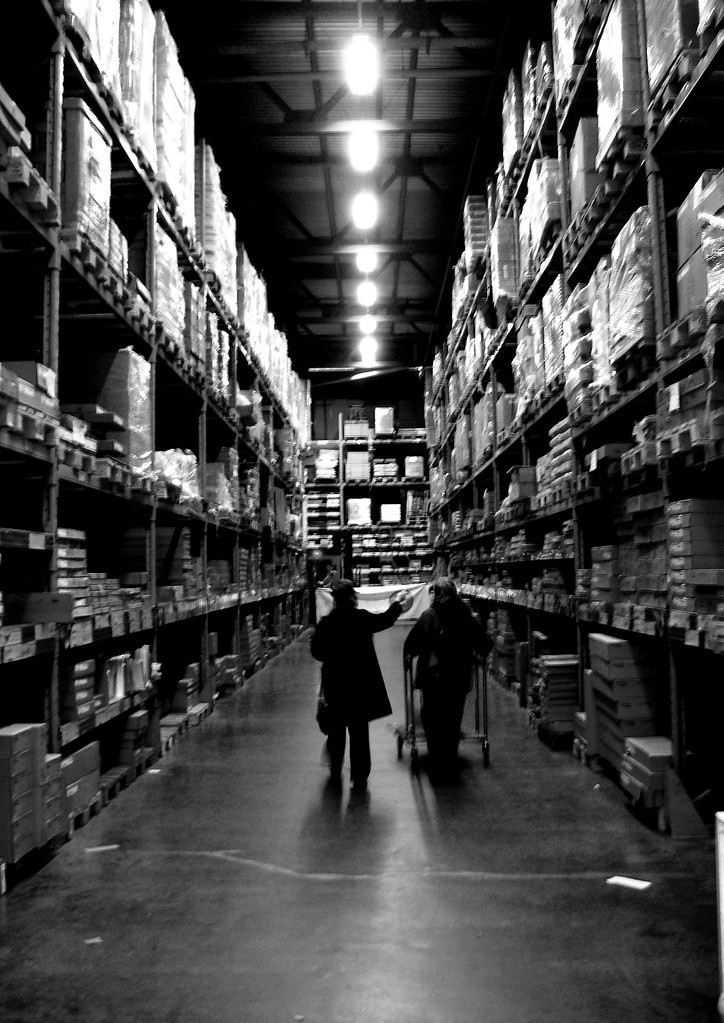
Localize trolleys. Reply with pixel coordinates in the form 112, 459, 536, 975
394, 649, 493, 767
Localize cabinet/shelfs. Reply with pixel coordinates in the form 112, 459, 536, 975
2, 0, 723, 898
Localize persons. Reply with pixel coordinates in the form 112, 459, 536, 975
310, 563, 341, 626
404, 578, 495, 781
311, 579, 414, 797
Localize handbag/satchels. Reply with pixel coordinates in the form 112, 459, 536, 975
316, 699, 334, 733
